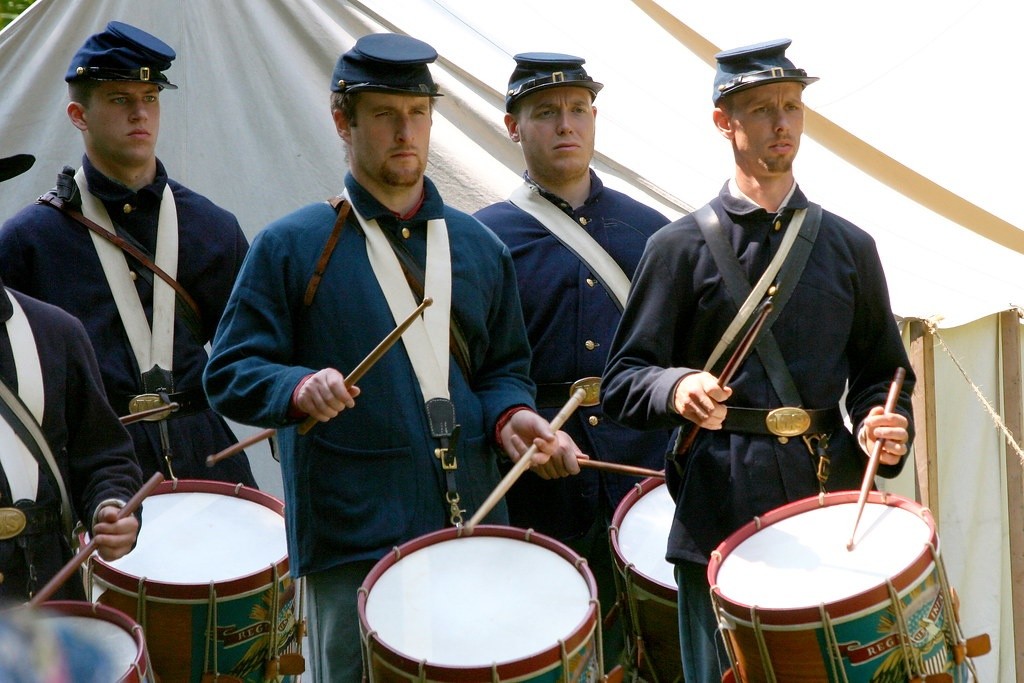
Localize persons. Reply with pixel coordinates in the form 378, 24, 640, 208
1, 21, 262, 498
1, 153, 148, 612
598, 33, 921, 683
462, 50, 676, 675
198, 30, 565, 683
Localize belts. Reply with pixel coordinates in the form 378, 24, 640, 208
535, 377, 601, 407
106, 388, 210, 422
721, 408, 845, 436
1, 503, 60, 540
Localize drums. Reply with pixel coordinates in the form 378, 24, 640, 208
605, 466, 687, 683
72, 475, 308, 683
703, 487, 995, 682
352, 517, 607, 683
0, 599, 164, 683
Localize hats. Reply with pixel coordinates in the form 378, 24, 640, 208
330, 34, 443, 97
506, 53, 604, 113
66, 21, 177, 92
712, 38, 820, 107
